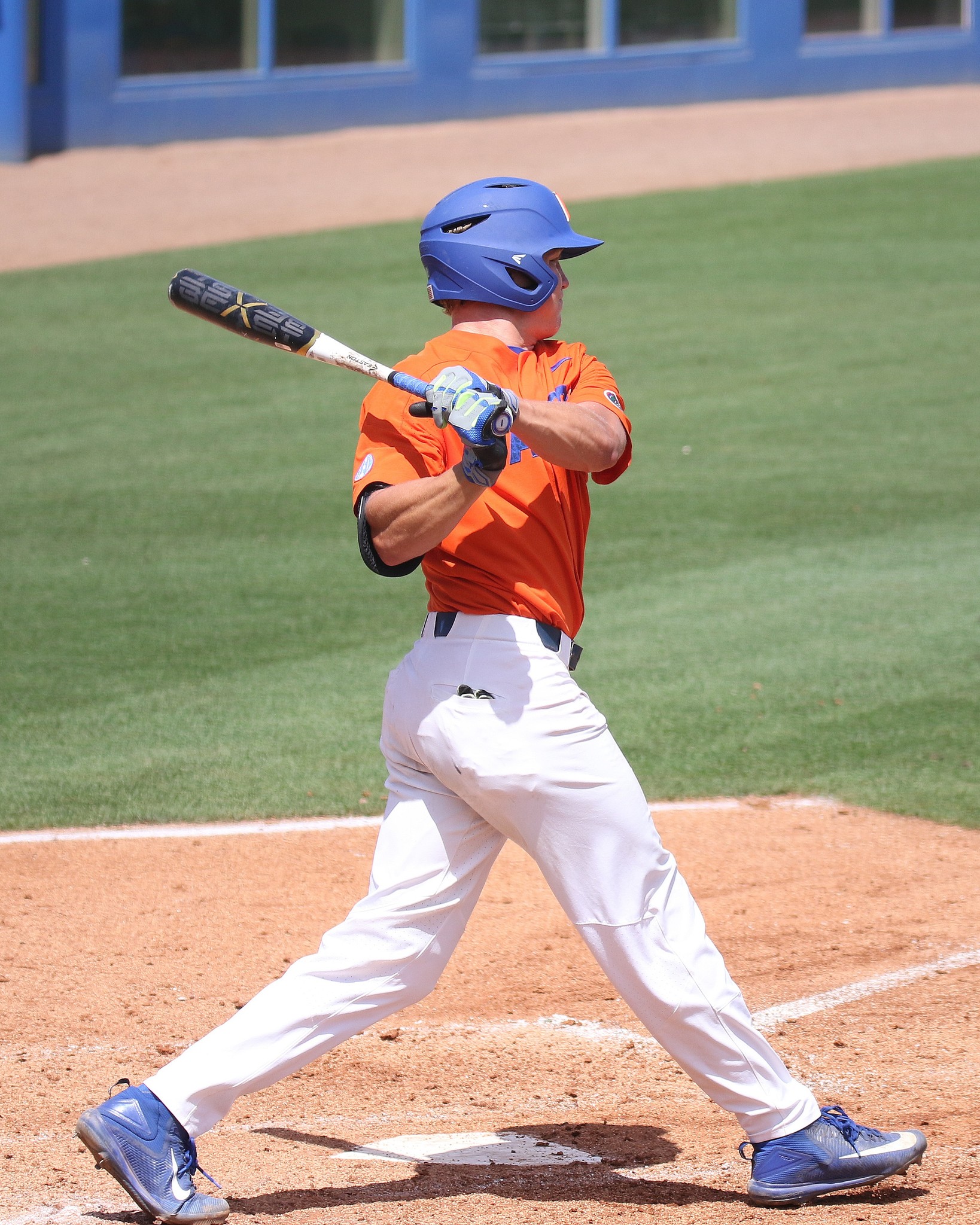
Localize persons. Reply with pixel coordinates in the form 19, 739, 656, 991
75, 169, 926, 1223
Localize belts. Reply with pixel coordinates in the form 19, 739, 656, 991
421, 611, 583, 671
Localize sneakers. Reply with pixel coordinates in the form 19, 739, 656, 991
73, 1078, 231, 1225
739, 1105, 928, 1208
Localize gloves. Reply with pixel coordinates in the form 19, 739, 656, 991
408, 365, 520, 429
448, 387, 508, 488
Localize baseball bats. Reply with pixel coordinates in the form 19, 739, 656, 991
168, 267, 514, 436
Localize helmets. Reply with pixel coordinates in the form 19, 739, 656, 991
419, 176, 605, 312
